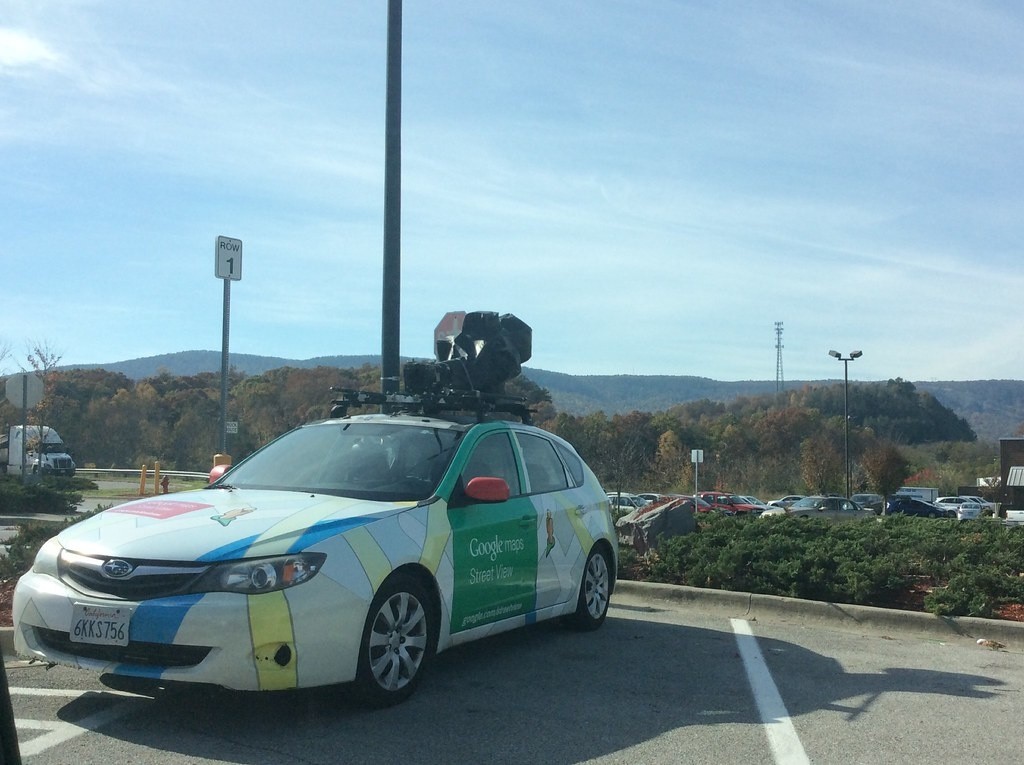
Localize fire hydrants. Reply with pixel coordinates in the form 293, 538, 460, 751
160, 476, 170, 494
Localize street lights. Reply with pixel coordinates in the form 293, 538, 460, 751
829, 350, 863, 498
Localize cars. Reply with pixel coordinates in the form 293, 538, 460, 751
605, 495, 1024, 527
11, 410, 621, 709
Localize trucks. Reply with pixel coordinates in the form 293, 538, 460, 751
1, 425, 77, 477
